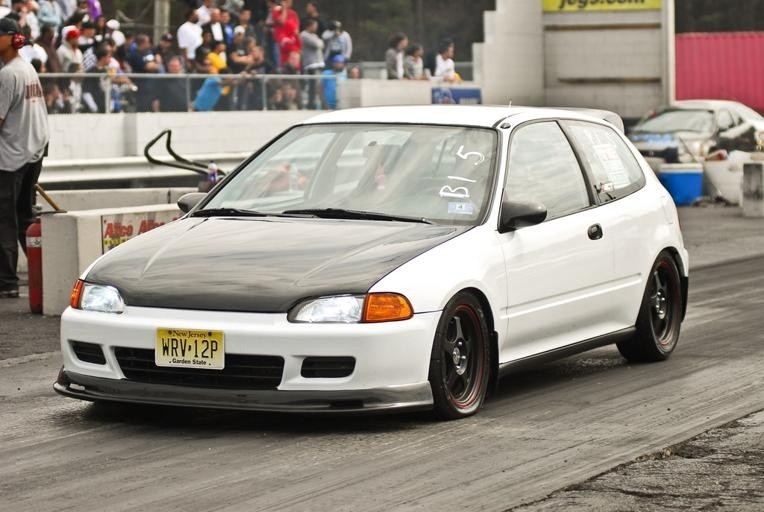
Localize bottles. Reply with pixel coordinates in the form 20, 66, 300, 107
208, 158, 216, 185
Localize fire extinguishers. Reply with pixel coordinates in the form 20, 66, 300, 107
26, 204, 67, 314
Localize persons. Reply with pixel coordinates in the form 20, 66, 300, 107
0, 0, 365, 113
403, 42, 429, 81
422, 37, 458, 83
0, 15, 53, 299
383, 31, 410, 80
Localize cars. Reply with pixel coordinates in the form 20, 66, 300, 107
48, 99, 693, 417
626, 99, 763, 162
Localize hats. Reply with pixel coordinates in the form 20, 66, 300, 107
235, 25, 245, 33
162, 33, 173, 40
107, 19, 121, 30
0, 18, 21, 35
111, 31, 126, 46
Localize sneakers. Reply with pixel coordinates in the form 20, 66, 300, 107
1, 288, 19, 299
